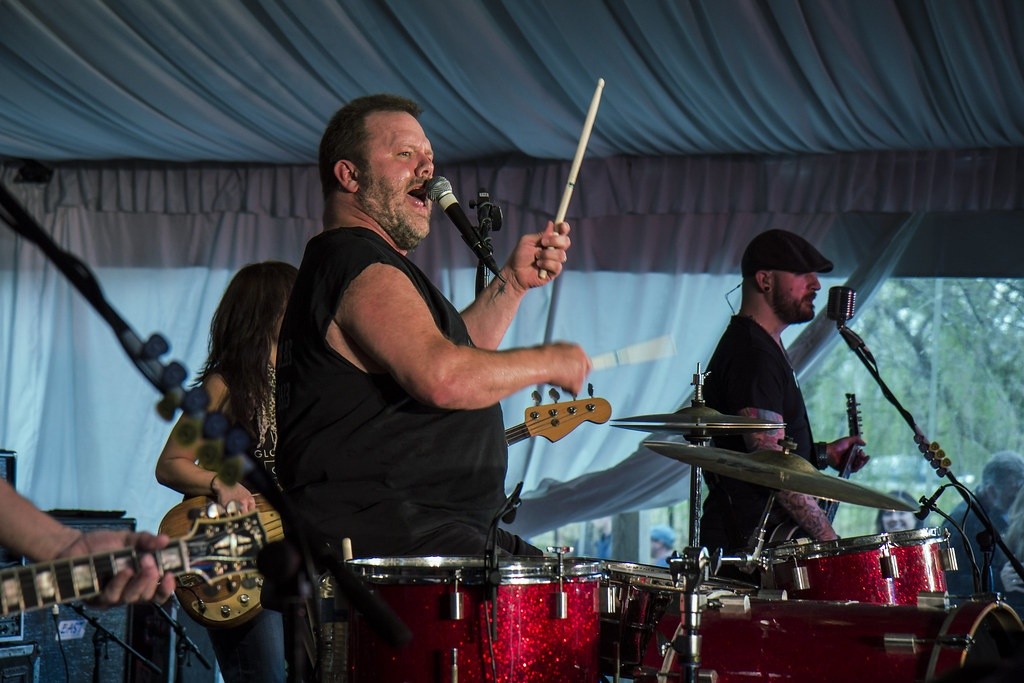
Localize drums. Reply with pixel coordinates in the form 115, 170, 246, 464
566, 557, 760, 680
761, 525, 960, 606
641, 593, 1024, 683
344, 556, 602, 683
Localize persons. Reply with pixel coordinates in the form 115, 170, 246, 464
875, 490, 923, 533
942, 450, 1024, 606
651, 526, 679, 566
155, 261, 300, 683
701, 228, 868, 580
275, 94, 590, 558
0, 478, 175, 609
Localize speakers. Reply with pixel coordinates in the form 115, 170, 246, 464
23, 518, 136, 683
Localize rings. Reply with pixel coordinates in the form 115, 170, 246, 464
1012, 579, 1018, 585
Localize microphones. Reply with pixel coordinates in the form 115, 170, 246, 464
914, 486, 944, 520
827, 286, 876, 363
425, 176, 501, 274
502, 482, 524, 524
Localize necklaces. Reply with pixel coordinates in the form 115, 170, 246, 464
754, 320, 798, 388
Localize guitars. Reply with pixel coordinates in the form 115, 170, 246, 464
768, 393, 863, 544
0, 498, 267, 619
157, 383, 612, 630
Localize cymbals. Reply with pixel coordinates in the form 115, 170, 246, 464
642, 442, 921, 514
609, 425, 786, 435
610, 407, 787, 426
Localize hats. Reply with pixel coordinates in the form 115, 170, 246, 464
741, 229, 834, 277
650, 526, 675, 549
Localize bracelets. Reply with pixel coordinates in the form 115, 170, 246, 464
818, 441, 827, 469
210, 475, 218, 494
